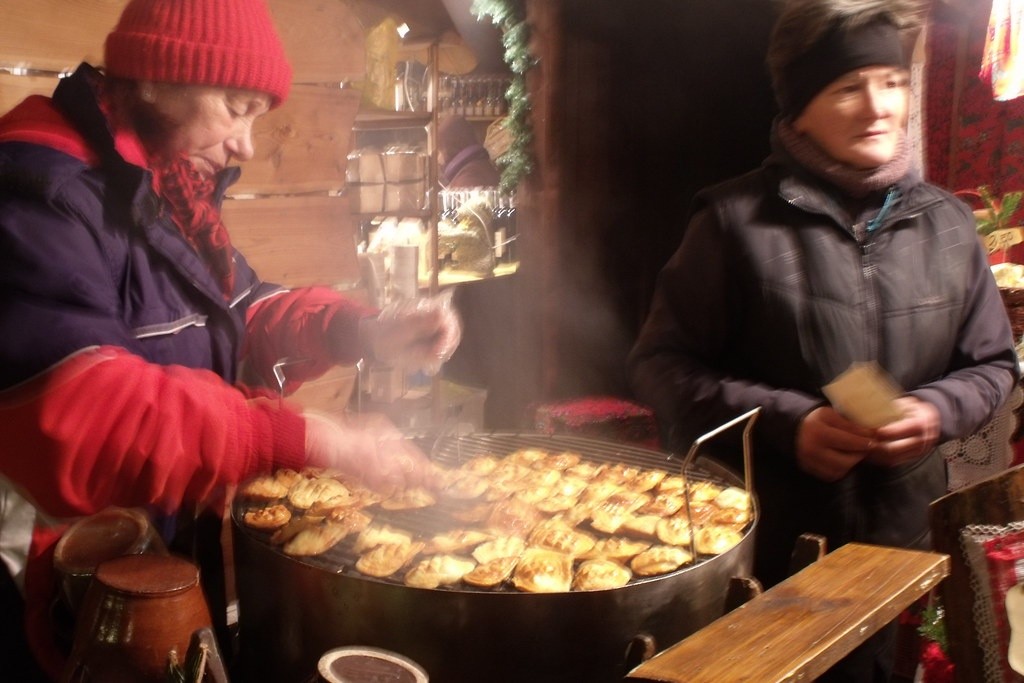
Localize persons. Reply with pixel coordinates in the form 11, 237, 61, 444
0, 0, 460, 683
624, 1, 1020, 683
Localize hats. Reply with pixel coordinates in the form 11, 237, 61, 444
103, 0, 291, 110
766, 0, 923, 124
